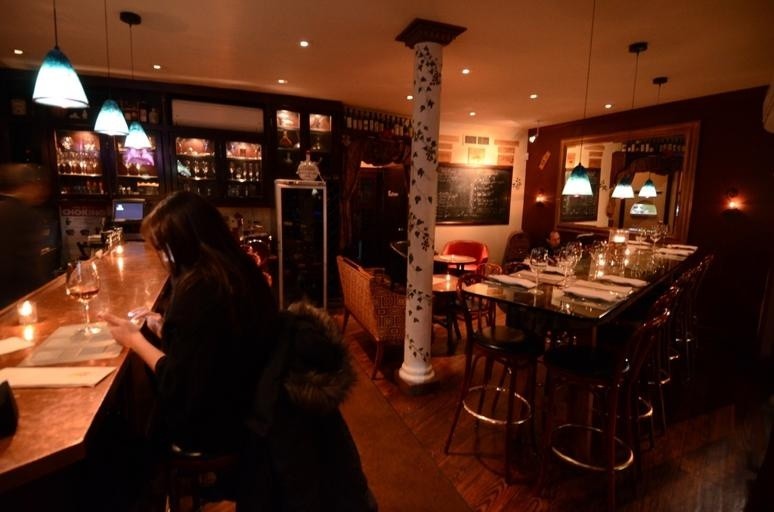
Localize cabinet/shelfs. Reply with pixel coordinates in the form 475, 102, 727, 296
33, 73, 344, 196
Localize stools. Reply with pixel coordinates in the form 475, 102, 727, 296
445, 325, 540, 479
537, 343, 634, 512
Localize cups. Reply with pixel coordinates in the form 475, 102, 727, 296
134, 160, 141, 175
182, 160, 215, 176
636, 225, 648, 255
123, 155, 132, 175
249, 163, 253, 180
65, 261, 100, 338
650, 222, 667, 255
229, 162, 234, 179
236, 167, 242, 179
528, 248, 547, 295
56, 136, 101, 173
255, 163, 260, 181
243, 163, 247, 180
558, 241, 582, 289
589, 239, 627, 275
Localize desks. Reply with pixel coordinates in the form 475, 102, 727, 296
432, 275, 467, 354
434, 254, 475, 272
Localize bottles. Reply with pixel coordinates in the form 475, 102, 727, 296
388, 116, 392, 133
184, 159, 209, 179
408, 119, 412, 138
378, 114, 383, 133
373, 113, 378, 133
56, 149, 97, 174
357, 110, 362, 132
368, 112, 373, 133
403, 119, 409, 136
363, 111, 368, 133
384, 115, 388, 131
279, 131, 292, 148
393, 117, 399, 137
352, 109, 357, 130
346, 108, 352, 129
399, 118, 403, 137
298, 151, 318, 180
228, 161, 259, 181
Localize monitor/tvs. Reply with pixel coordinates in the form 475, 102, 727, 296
112, 199, 146, 223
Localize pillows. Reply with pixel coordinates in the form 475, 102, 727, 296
376, 272, 393, 286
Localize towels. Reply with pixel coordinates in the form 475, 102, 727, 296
598, 274, 648, 288
657, 248, 694, 257
666, 244, 698, 251
652, 253, 687, 261
563, 286, 625, 305
489, 274, 536, 290
575, 279, 632, 295
528, 259, 573, 276
509, 270, 564, 286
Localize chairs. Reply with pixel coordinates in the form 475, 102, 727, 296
650, 285, 685, 424
476, 263, 501, 327
454, 274, 490, 340
617, 305, 671, 476
694, 254, 715, 342
503, 232, 531, 264
669, 269, 701, 381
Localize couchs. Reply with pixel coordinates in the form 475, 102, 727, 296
444, 241, 488, 275
336, 256, 406, 381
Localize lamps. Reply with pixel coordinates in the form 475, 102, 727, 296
562, 0, 595, 195
119, 10, 152, 150
638, 76, 670, 198
529, 120, 540, 144
34, 1, 86, 109
94, 0, 128, 137
612, 42, 648, 201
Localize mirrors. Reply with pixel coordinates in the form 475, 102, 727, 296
277, 179, 327, 311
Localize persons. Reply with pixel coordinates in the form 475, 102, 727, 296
536, 231, 563, 267
96, 192, 278, 511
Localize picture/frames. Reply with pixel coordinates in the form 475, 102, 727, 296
554, 117, 700, 245
437, 163, 513, 226
